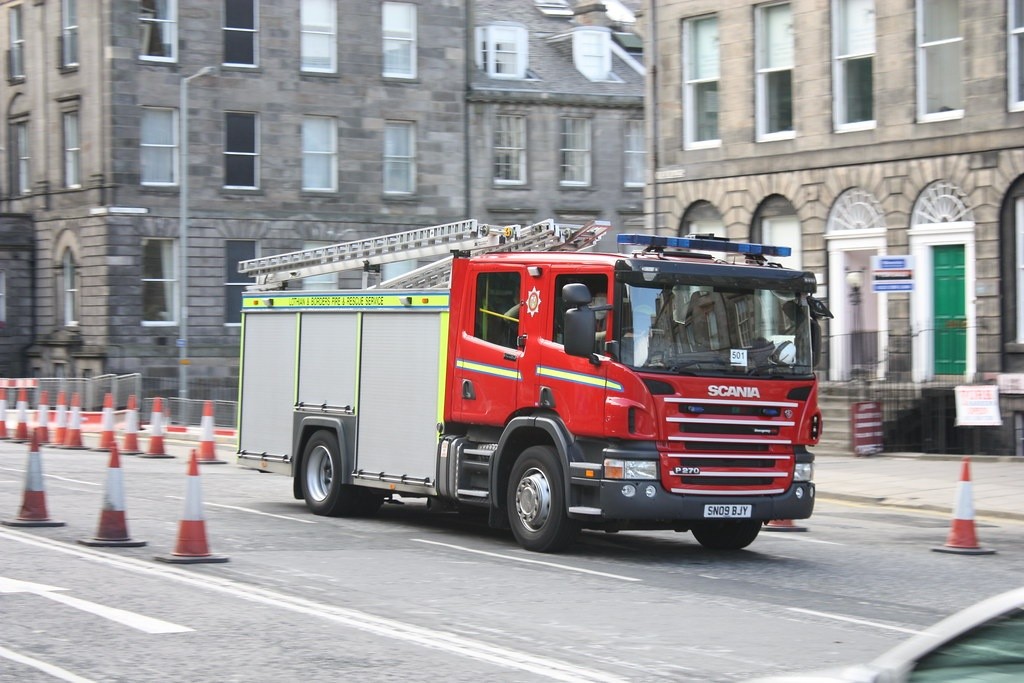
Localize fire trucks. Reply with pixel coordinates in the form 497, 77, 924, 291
233, 217, 837, 556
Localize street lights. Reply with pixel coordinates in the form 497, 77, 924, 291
175, 64, 223, 412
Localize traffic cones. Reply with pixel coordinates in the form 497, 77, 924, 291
22, 390, 53, 445
0, 388, 14, 440
42, 389, 68, 448
57, 393, 90, 451
6, 388, 33, 443
116, 393, 148, 456
87, 393, 121, 452
185, 399, 229, 464
930, 456, 997, 555
0, 428, 68, 528
135, 396, 174, 460
76, 441, 146, 549
149, 448, 231, 564
759, 518, 808, 533
